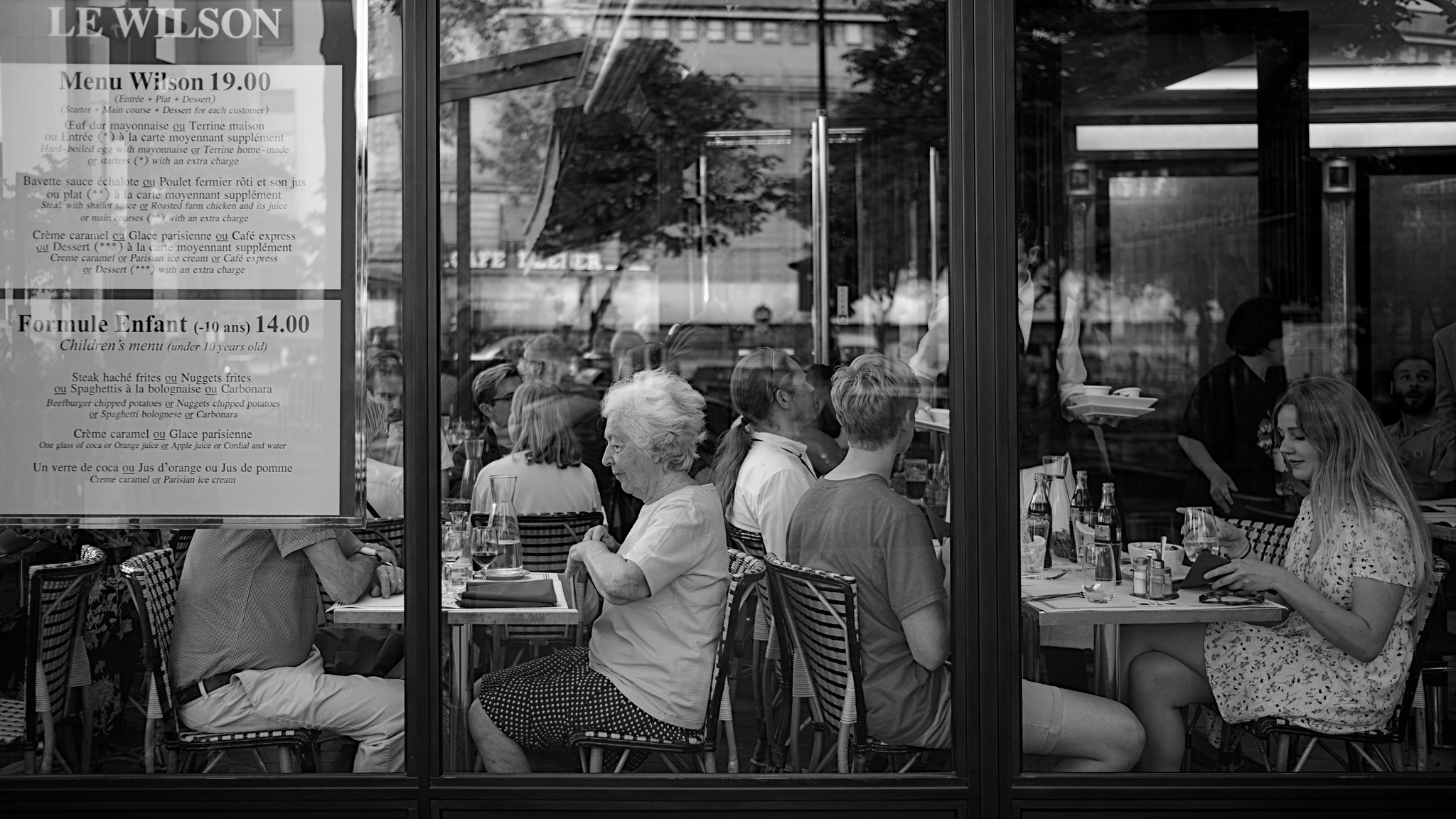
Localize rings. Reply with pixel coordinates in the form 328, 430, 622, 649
397, 579, 404, 583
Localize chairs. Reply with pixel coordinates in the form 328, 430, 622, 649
0, 510, 924, 776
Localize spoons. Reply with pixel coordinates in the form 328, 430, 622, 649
1021, 584, 1100, 603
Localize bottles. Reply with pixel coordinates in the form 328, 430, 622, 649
441, 415, 484, 503
1094, 482, 1122, 586
486, 474, 523, 575
1042, 456, 1069, 533
1133, 556, 1173, 600
1069, 470, 1094, 563
1027, 472, 1052, 570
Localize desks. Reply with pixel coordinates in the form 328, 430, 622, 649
1021, 565, 1289, 702
333, 573, 579, 769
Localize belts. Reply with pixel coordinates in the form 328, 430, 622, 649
176, 666, 235, 706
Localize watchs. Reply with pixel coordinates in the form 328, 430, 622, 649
352, 546, 392, 566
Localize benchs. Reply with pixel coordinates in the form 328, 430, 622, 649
1220, 516, 1456, 772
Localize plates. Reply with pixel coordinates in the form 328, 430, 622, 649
473, 568, 531, 580
915, 420, 950, 435
1119, 565, 1193, 582
1066, 393, 1159, 422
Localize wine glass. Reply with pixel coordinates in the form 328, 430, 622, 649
442, 524, 464, 598
471, 527, 499, 580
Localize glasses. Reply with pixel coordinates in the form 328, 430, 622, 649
753, 345, 780, 399
486, 392, 515, 403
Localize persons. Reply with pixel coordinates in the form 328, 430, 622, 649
913, 217, 1121, 503
361, 297, 1456, 672
469, 366, 732, 776
0, 524, 156, 775
1110, 374, 1436, 774
787, 352, 1149, 775
170, 528, 407, 773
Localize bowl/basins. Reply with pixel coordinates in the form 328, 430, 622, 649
1128, 542, 1185, 577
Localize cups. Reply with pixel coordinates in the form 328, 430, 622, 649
891, 458, 947, 507
1185, 507, 1219, 562
930, 407, 950, 425
1083, 385, 1112, 394
1020, 519, 1049, 579
1114, 387, 1141, 397
1072, 511, 1098, 565
442, 511, 474, 593
1081, 543, 1117, 604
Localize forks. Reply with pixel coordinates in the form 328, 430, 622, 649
1021, 568, 1071, 580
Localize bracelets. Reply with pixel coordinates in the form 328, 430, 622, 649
1230, 539, 1250, 558
1227, 542, 1252, 561
